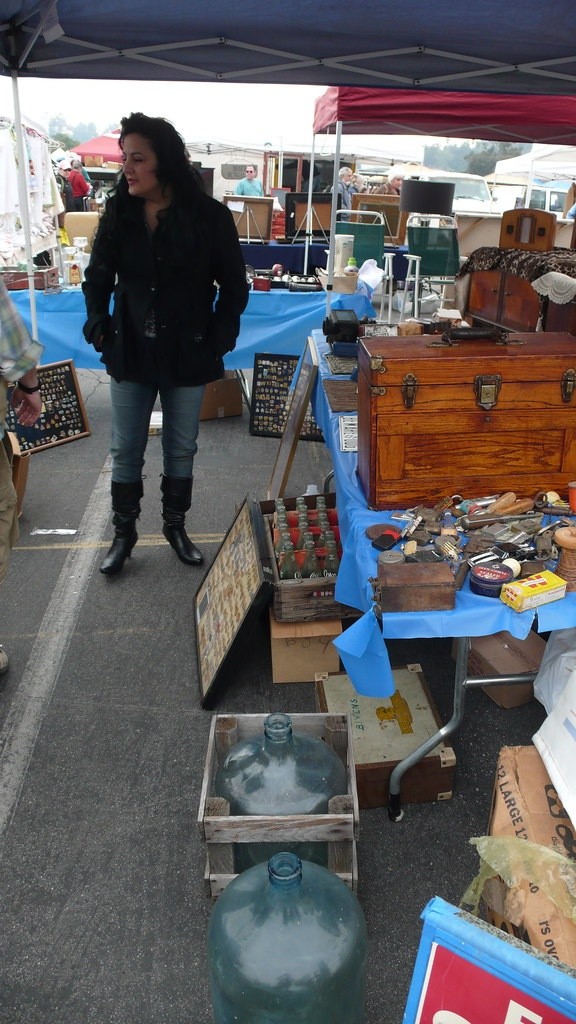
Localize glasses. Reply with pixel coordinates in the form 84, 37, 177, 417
246, 171, 252, 173
64, 168, 71, 171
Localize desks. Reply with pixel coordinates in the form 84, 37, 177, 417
300, 317, 576, 834
241, 245, 408, 286
5, 272, 380, 424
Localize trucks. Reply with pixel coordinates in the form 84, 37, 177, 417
184, 141, 576, 245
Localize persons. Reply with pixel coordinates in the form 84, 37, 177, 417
371, 170, 406, 194
331, 167, 352, 222
235, 166, 263, 196
348, 174, 363, 204
81, 112, 251, 574
28, 159, 92, 213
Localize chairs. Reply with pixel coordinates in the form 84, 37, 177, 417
398, 211, 463, 319
322, 206, 399, 325
64, 211, 103, 252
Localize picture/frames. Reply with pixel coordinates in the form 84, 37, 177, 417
5, 355, 91, 456
347, 193, 407, 248
221, 194, 275, 245
265, 335, 319, 502
184, 491, 278, 706
250, 350, 325, 443
282, 191, 345, 241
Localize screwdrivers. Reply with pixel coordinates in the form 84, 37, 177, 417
460, 514, 543, 530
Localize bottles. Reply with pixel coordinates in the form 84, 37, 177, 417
301, 484, 320, 496
204, 851, 369, 1024
344, 257, 359, 273
273, 496, 340, 579
62, 237, 91, 289
213, 711, 348, 874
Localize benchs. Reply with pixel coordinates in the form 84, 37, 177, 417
270, 276, 290, 288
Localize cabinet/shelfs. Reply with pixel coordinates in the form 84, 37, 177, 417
464, 246, 576, 332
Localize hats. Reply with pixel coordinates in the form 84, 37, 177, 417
59, 160, 71, 169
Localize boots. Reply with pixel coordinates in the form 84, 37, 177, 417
100, 475, 146, 574
160, 476, 203, 564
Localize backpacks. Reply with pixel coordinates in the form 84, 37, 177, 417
323, 182, 344, 192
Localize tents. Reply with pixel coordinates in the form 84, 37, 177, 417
1, 0, 576, 367
68, 125, 125, 163
303, 86, 576, 316
1, 115, 66, 282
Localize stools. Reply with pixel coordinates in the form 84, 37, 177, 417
254, 268, 272, 276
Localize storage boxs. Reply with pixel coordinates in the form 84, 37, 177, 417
448, 630, 552, 709
484, 744, 576, 969
497, 209, 557, 251
501, 567, 568, 614
192, 707, 366, 904
259, 490, 365, 623
377, 562, 455, 613
84, 155, 103, 167
198, 369, 241, 423
289, 273, 321, 291
317, 266, 361, 295
0, 262, 60, 291
309, 654, 467, 813
354, 327, 576, 509
266, 601, 344, 686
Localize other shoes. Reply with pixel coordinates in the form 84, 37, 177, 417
0, 645, 9, 673
